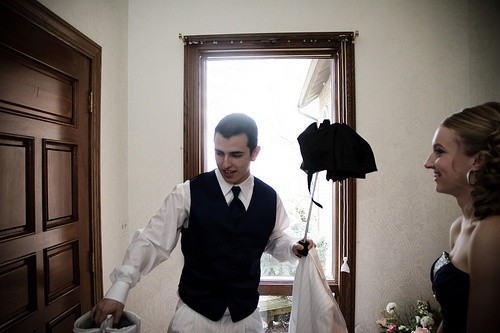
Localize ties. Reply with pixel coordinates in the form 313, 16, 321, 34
229, 186, 246, 218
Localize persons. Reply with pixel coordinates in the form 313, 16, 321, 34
422, 100, 500, 333
90, 112, 317, 333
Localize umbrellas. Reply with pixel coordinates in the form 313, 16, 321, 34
295, 119, 378, 258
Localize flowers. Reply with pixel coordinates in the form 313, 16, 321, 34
375, 297, 440, 333
